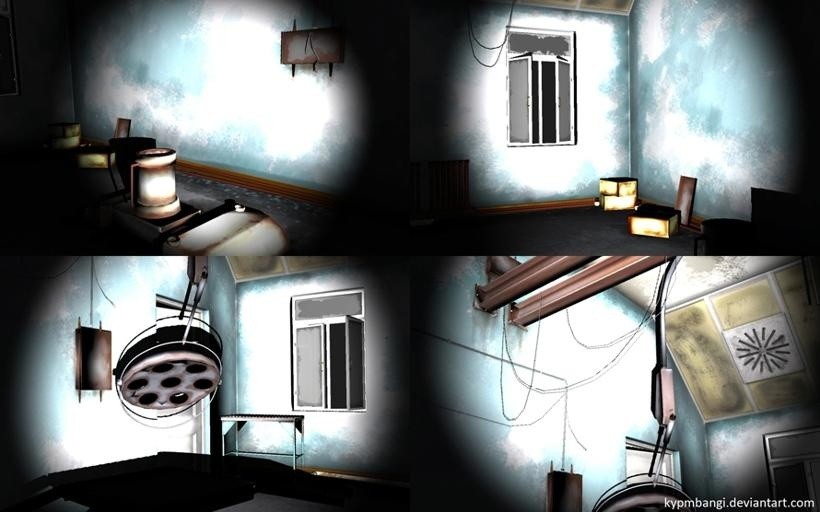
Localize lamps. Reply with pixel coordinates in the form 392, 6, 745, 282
597, 176, 639, 211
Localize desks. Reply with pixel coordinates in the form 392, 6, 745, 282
220, 413, 307, 470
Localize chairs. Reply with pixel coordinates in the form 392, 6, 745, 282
694, 188, 801, 256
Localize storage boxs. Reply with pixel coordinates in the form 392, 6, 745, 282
627, 204, 681, 239
